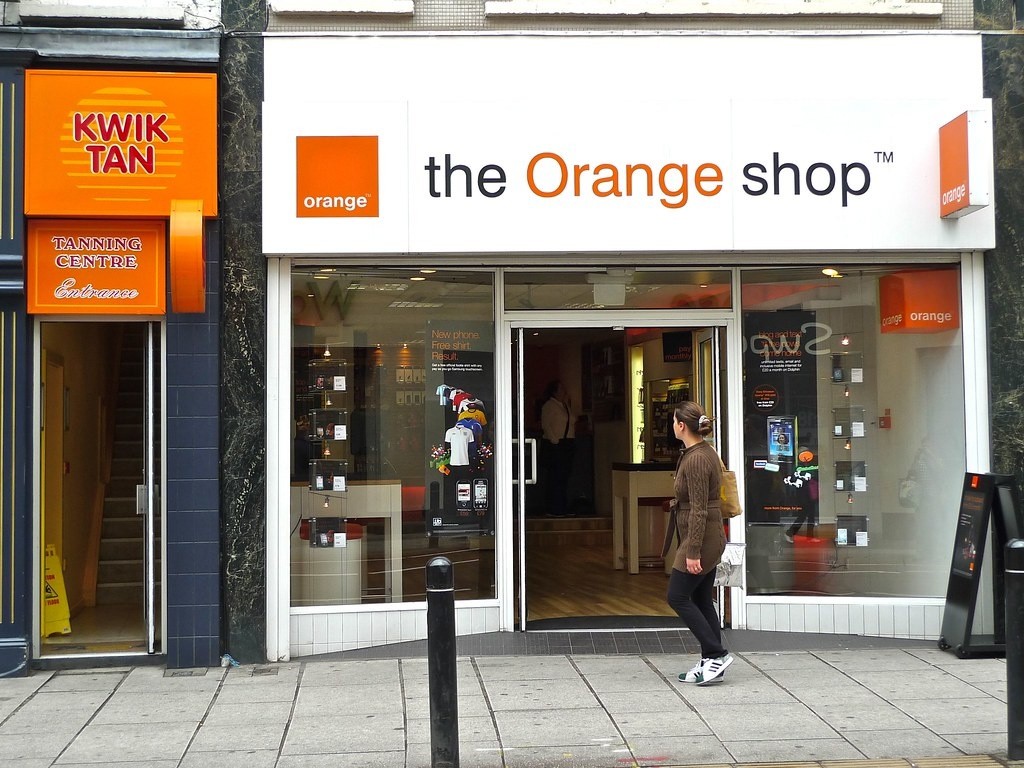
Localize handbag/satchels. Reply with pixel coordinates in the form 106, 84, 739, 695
708, 443, 742, 520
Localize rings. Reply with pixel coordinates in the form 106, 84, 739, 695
694, 567, 698, 571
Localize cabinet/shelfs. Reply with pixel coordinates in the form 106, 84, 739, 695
380, 366, 425, 480
591, 336, 627, 423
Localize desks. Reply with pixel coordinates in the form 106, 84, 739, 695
610, 461, 679, 575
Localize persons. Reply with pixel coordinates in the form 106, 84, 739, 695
776, 434, 788, 444
660, 400, 735, 686
782, 434, 822, 545
537, 381, 581, 519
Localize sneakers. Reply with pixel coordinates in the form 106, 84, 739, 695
694, 653, 734, 685
677, 661, 725, 684
